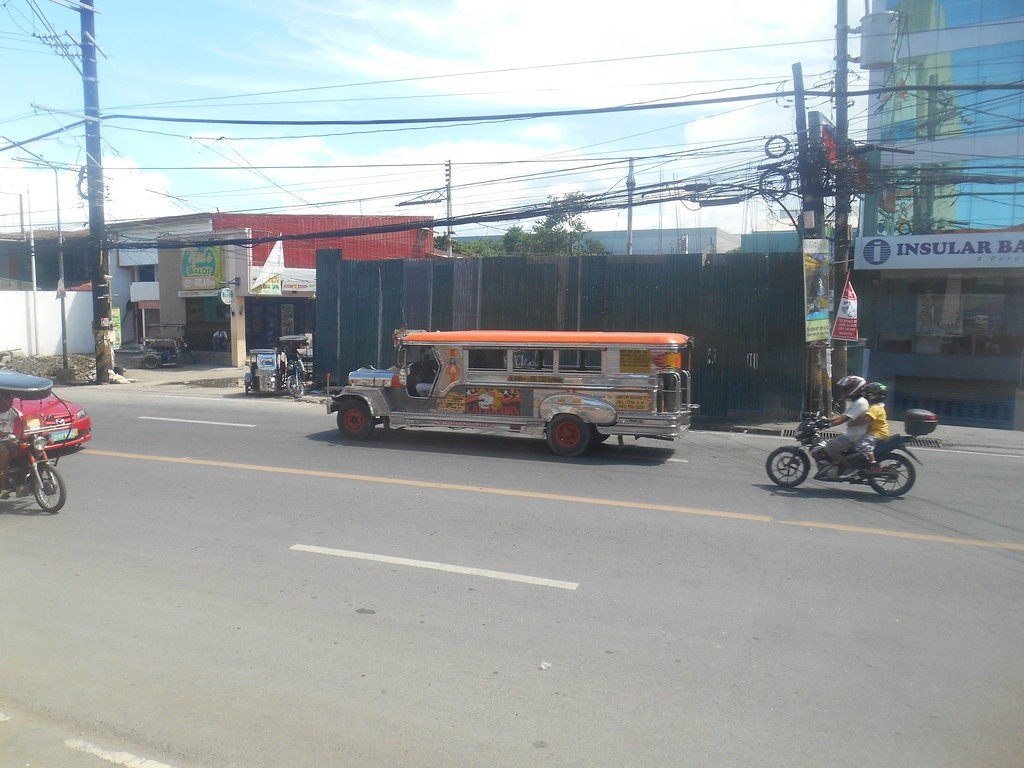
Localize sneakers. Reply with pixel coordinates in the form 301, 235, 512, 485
863, 463, 882, 474
839, 467, 859, 479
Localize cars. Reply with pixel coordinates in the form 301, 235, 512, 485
10, 396, 92, 457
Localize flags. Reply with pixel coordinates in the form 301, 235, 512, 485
830, 273, 858, 341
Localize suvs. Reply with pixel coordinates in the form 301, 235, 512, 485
326, 327, 700, 460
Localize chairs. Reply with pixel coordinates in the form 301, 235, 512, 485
416, 382, 434, 397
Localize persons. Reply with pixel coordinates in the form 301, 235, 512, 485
212, 325, 227, 341
0, 394, 23, 500
414, 349, 434, 397
818, 376, 889, 479
283, 345, 307, 381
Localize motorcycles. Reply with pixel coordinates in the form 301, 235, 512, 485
0, 369, 68, 514
140, 324, 193, 369
766, 403, 938, 496
243, 332, 309, 400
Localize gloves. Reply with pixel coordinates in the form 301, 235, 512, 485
817, 422, 829, 431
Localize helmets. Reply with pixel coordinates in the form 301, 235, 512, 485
836, 375, 867, 398
864, 382, 888, 402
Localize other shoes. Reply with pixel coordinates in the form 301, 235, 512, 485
0, 489, 12, 500
